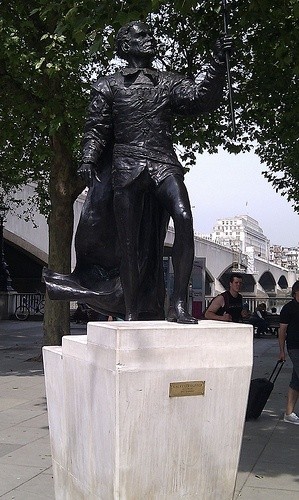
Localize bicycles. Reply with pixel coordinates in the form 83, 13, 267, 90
14, 293, 46, 321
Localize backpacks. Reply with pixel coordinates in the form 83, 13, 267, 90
200, 291, 243, 320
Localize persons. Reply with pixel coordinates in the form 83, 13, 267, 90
249, 303, 280, 338
277, 280, 299, 426
39, 21, 235, 325
204, 273, 248, 322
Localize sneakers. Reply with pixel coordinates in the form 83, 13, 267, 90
284, 411, 299, 426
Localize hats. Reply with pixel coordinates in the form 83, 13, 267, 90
271, 308, 276, 311
259, 303, 266, 310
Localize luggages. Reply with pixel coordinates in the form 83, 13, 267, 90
245, 359, 286, 420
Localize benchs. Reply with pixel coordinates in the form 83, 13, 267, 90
241, 315, 281, 338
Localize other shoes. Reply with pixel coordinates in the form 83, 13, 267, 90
257, 333, 262, 336
262, 333, 266, 335
